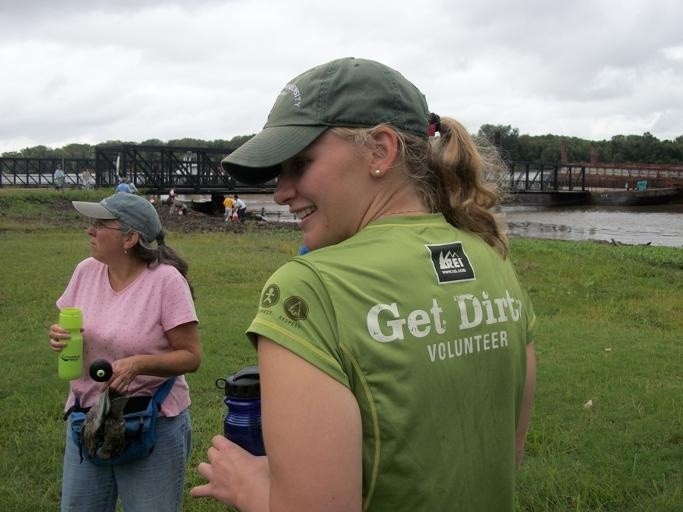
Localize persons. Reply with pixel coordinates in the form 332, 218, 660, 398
189, 58, 535, 510
178, 204, 188, 216
127, 178, 138, 192
149, 195, 159, 210
54, 164, 65, 190
168, 186, 177, 215
116, 178, 135, 193
82, 167, 91, 191
49, 193, 198, 511
222, 193, 247, 224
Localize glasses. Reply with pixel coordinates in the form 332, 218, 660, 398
91, 218, 126, 234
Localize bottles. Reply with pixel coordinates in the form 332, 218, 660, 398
57, 308, 83, 381
223, 369, 266, 456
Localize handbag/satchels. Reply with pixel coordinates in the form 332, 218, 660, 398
64, 392, 164, 471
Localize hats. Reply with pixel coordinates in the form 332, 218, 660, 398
67, 189, 163, 244
217, 55, 433, 189
